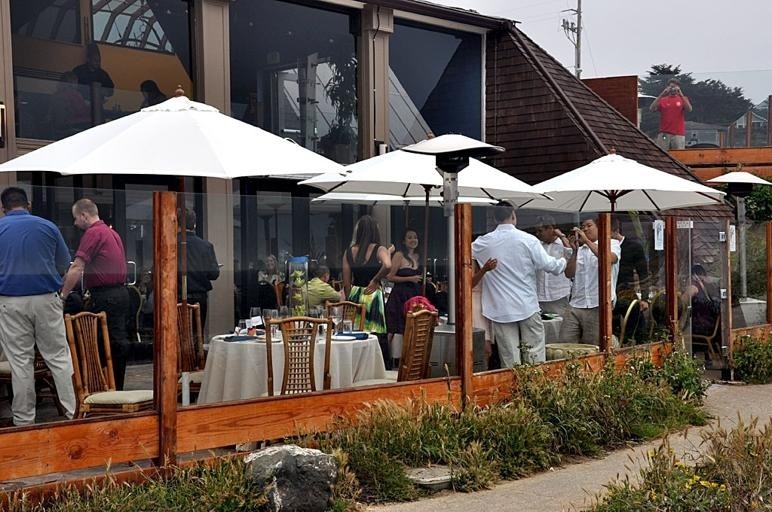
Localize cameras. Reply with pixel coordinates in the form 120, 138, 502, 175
670, 88, 678, 93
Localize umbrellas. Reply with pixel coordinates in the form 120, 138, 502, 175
0, 92, 343, 407
311, 190, 500, 231
508, 152, 727, 217
299, 132, 544, 309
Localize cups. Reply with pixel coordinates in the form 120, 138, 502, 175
438, 316, 449, 326
342, 320, 352, 335
238, 318, 247, 334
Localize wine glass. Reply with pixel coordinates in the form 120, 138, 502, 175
331, 306, 343, 335
248, 306, 287, 338
307, 305, 328, 342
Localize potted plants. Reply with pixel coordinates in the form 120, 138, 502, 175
320, 54, 362, 164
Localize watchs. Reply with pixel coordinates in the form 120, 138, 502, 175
560, 234, 566, 238
60, 293, 66, 301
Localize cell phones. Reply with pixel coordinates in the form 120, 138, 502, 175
570, 230, 578, 240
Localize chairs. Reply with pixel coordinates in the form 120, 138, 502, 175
683, 311, 721, 370
275, 280, 285, 309
0, 350, 64, 417
64, 311, 154, 468
619, 299, 641, 347
167, 301, 437, 446
648, 290, 683, 339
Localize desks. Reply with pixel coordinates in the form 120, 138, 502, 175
438, 327, 486, 377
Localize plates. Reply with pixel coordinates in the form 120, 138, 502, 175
257, 337, 282, 342
331, 335, 357, 341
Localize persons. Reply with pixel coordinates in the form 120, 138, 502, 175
681, 265, 722, 370
471, 251, 497, 371
471, 200, 569, 372
257, 255, 285, 310
560, 217, 622, 345
60, 197, 129, 391
51, 71, 109, 130
297, 266, 341, 322
386, 228, 432, 368
140, 79, 167, 110
145, 208, 220, 370
533, 214, 574, 318
342, 215, 392, 370
71, 44, 114, 107
1, 187, 79, 425
650, 78, 693, 150
612, 220, 650, 347
